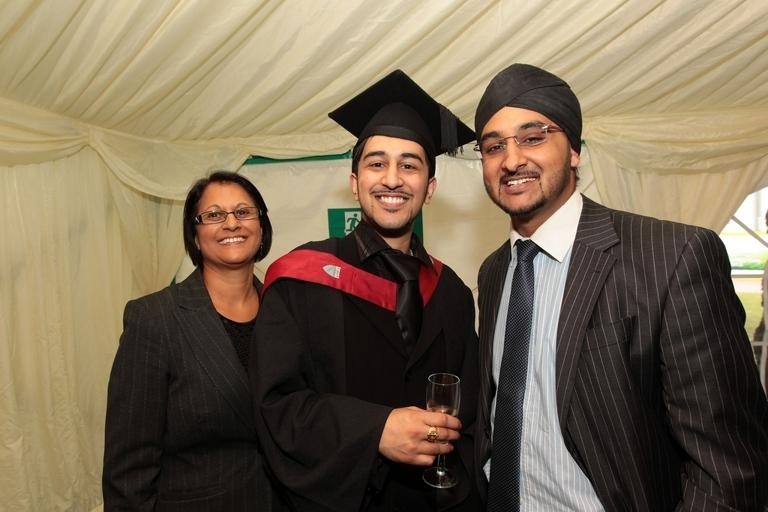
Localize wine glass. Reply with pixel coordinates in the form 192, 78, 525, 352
421, 372, 463, 490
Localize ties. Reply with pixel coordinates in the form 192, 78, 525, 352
492, 242, 538, 512
385, 250, 426, 361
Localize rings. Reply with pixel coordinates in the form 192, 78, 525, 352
426, 427, 439, 442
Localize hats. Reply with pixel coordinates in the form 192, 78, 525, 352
475, 63, 584, 154
328, 66, 475, 179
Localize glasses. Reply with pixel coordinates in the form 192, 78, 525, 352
479, 123, 567, 154
188, 202, 264, 226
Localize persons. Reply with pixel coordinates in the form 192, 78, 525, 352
474, 62, 765, 510
245, 125, 485, 508
100, 169, 272, 509
